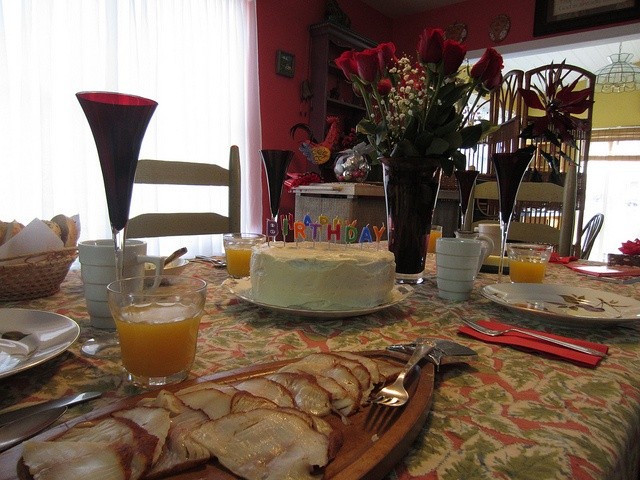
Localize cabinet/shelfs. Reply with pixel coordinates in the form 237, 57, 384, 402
309, 22, 384, 145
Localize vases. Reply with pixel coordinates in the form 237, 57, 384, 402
374, 154, 446, 286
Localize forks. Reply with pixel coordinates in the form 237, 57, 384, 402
362, 340, 437, 435
460, 316, 608, 359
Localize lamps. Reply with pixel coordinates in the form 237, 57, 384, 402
586, 53, 640, 95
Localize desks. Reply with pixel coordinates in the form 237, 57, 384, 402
286, 181, 460, 242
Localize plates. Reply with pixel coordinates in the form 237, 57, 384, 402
481, 283, 640, 327
0, 401, 67, 453
222, 275, 417, 318
1, 308, 80, 379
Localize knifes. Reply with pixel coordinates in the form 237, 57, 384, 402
0, 390, 103, 427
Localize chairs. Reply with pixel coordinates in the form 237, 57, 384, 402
463, 164, 577, 259
577, 211, 605, 258
126, 144, 240, 254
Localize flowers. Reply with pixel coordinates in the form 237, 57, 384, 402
334, 26, 503, 164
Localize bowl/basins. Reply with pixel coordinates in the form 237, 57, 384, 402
144, 258, 190, 285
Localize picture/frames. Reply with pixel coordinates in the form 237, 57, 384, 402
275, 48, 297, 78
532, 1, 640, 37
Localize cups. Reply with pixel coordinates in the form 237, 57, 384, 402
427, 225, 443, 254
79, 240, 147, 330
224, 233, 267, 279
436, 238, 481, 301
454, 230, 494, 277
106, 276, 208, 386
474, 223, 501, 257
506, 243, 554, 283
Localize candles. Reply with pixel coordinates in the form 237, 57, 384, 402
344, 219, 357, 236
308, 222, 322, 239
332, 216, 343, 231
280, 213, 295, 232
302, 214, 314, 230
344, 225, 359, 243
265, 219, 278, 237
281, 217, 288, 237
372, 222, 386, 244
326, 223, 341, 241
317, 214, 329, 233
293, 221, 307, 241
358, 223, 373, 244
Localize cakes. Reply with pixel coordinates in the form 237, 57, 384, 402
249, 240, 396, 313
